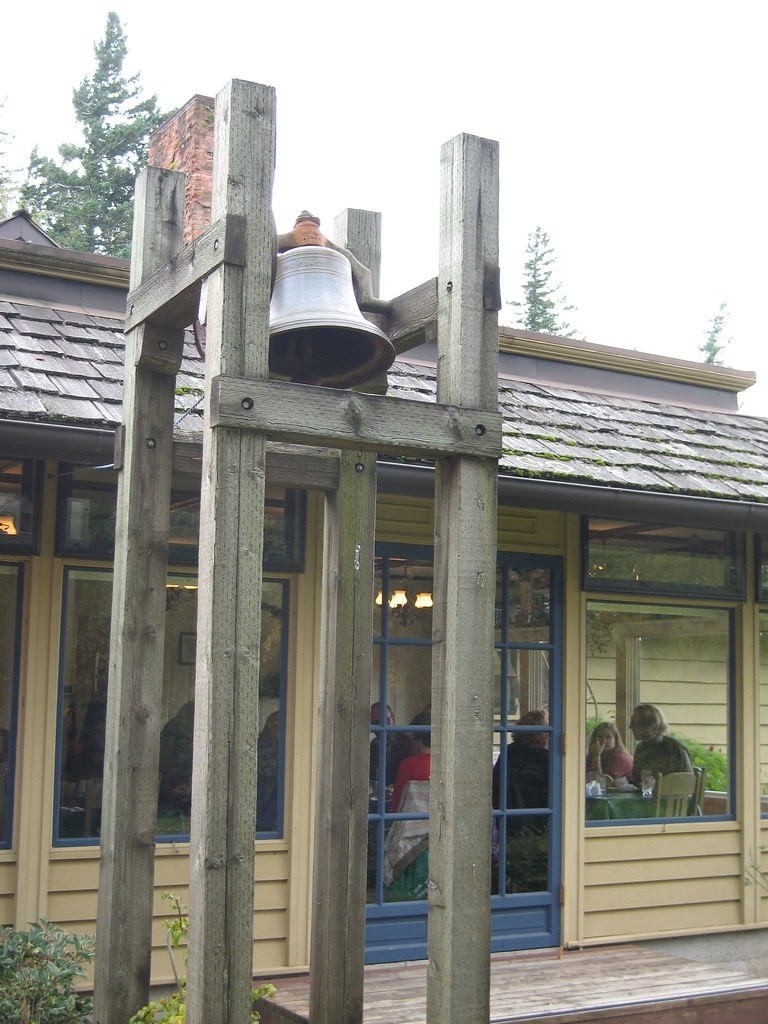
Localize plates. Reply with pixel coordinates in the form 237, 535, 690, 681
607, 786, 637, 793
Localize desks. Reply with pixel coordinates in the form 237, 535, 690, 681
586, 788, 696, 821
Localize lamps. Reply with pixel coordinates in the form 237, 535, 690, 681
0, 511, 17, 537
166, 585, 198, 612
374, 566, 434, 626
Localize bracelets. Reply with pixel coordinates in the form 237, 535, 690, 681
596, 753, 601, 756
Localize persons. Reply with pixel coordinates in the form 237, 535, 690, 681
630, 704, 702, 816
492, 709, 550, 836
585, 721, 634, 782
369, 702, 431, 827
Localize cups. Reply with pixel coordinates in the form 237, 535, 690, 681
370, 780, 379, 797
586, 780, 601, 796
640, 770, 652, 797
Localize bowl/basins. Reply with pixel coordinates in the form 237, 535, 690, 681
614, 776, 628, 787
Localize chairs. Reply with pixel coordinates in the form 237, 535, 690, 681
653, 765, 709, 818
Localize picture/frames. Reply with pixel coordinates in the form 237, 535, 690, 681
178, 632, 197, 665
